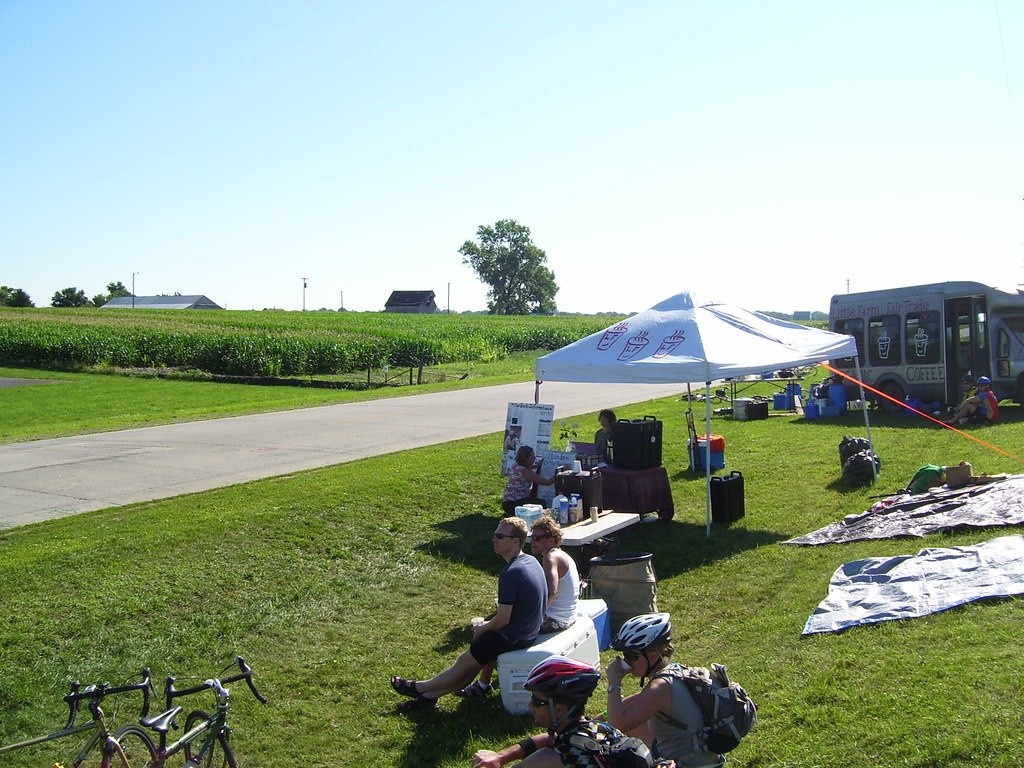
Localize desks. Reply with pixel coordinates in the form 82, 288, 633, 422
583, 466, 675, 529
525, 511, 642, 597
721, 377, 805, 415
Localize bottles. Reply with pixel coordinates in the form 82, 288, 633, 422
552, 492, 568, 508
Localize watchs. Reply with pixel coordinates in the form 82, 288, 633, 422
607, 685, 620, 692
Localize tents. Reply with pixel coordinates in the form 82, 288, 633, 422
534, 290, 877, 537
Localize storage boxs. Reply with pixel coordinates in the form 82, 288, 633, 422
577, 598, 613, 652
515, 504, 544, 532
761, 372, 773, 379
945, 463, 972, 489
688, 433, 726, 470
773, 392, 791, 410
762, 400, 774, 410
809, 383, 847, 412
747, 403, 768, 420
497, 617, 600, 717
822, 406, 844, 417
561, 451, 577, 470
731, 397, 756, 422
543, 450, 562, 467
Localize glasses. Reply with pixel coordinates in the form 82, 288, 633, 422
494, 531, 516, 540
530, 692, 568, 708
622, 650, 642, 663
532, 534, 549, 541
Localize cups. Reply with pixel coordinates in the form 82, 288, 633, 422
569, 503, 578, 523
573, 460, 582, 473
559, 499, 569, 525
619, 659, 633, 671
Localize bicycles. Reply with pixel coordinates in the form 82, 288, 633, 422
0, 656, 266, 768
681, 386, 732, 403
784, 364, 819, 377
753, 388, 810, 402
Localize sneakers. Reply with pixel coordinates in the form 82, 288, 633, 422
455, 680, 494, 697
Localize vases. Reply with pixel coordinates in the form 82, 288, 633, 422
565, 438, 573, 453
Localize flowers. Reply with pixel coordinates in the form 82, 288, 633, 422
558, 418, 580, 440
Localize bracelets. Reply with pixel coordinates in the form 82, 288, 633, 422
517, 737, 537, 756
601, 710, 608, 719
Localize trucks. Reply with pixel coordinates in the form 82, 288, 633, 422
829, 280, 1024, 414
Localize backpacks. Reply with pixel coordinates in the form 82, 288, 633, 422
907, 464, 945, 495
648, 663, 759, 754
557, 721, 676, 768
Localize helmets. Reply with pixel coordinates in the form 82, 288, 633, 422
977, 376, 991, 384
610, 613, 672, 651
524, 654, 601, 700
961, 375, 975, 382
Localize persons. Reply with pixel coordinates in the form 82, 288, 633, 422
471, 654, 627, 768
390, 516, 552, 710
595, 612, 726, 768
1011, 371, 1024, 418
501, 445, 555, 517
939, 374, 999, 428
456, 516, 579, 697
595, 409, 616, 458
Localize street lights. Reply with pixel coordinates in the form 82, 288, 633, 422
302, 277, 309, 310
133, 271, 140, 308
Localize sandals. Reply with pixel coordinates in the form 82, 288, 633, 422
391, 675, 426, 699
397, 696, 438, 713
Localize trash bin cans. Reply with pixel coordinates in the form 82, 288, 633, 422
590, 552, 659, 644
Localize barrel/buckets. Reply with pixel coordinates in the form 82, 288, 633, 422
802, 381, 848, 419
773, 383, 803, 411
